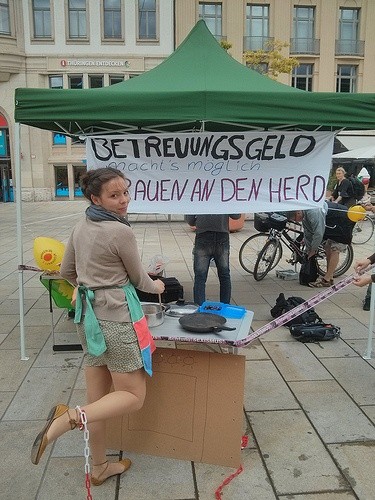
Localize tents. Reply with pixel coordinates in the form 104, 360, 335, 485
13, 18, 375, 363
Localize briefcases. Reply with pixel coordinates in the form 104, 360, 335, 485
135, 273, 184, 303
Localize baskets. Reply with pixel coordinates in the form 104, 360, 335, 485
254, 212, 271, 232
269, 212, 288, 232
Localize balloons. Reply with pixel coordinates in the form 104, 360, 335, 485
345, 207, 367, 222
32, 236, 67, 271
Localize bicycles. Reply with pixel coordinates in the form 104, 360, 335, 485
239, 212, 375, 282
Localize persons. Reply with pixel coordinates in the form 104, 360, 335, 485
183, 214, 243, 307
28, 165, 167, 487
329, 165, 357, 209
353, 249, 375, 288
288, 199, 356, 290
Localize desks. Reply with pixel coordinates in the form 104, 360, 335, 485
140, 302, 253, 354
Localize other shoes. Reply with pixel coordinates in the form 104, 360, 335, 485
308, 278, 334, 288
90, 458, 131, 486
30, 404, 79, 465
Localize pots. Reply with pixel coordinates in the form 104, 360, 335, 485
141, 303, 171, 328
178, 313, 236, 333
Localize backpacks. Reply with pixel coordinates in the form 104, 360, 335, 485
339, 175, 365, 200
